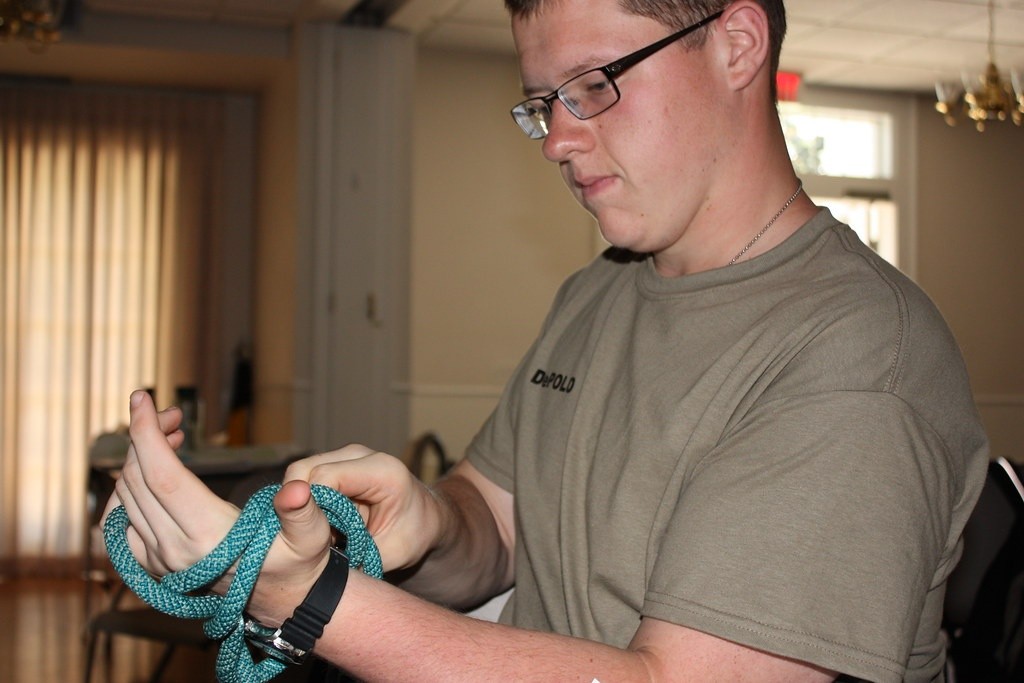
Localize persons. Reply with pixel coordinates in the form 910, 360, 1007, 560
97, 0, 989, 681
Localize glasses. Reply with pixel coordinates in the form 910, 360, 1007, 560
509, 11, 722, 140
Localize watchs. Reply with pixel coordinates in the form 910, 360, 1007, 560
242, 545, 350, 669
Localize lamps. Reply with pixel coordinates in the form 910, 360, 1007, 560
931, 0, 1024, 134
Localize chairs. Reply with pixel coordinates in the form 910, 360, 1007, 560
74, 418, 316, 683
